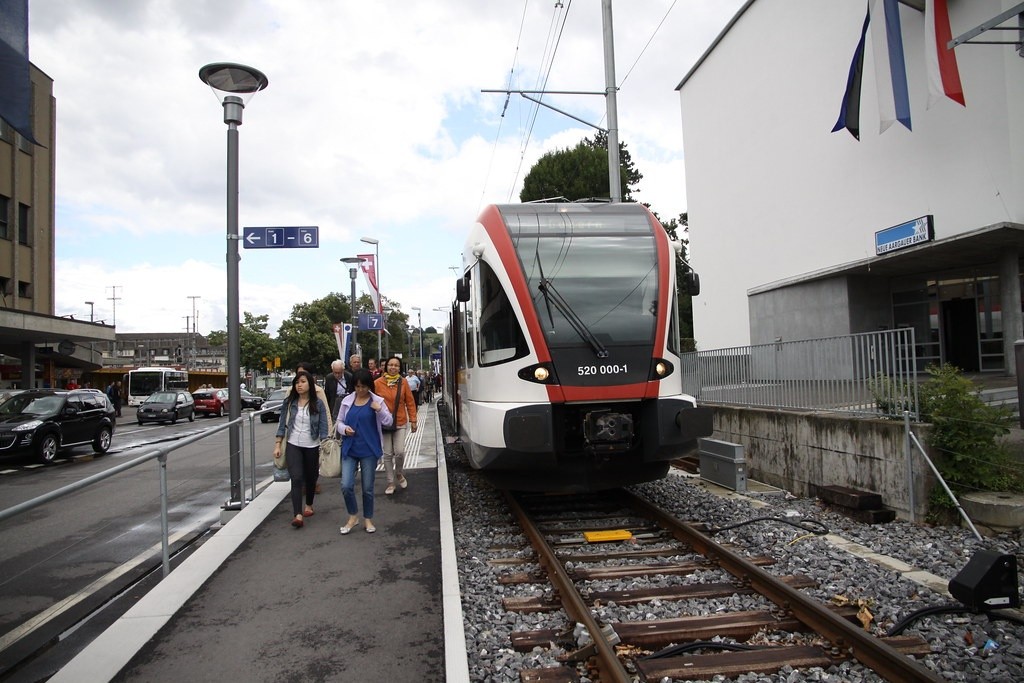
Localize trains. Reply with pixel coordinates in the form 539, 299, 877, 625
442, 201, 713, 491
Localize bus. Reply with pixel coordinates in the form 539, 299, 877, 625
122, 367, 189, 407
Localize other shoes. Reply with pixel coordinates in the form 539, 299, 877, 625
303, 505, 314, 517
398, 475, 407, 489
291, 519, 304, 528
340, 519, 360, 534
366, 527, 376, 532
384, 485, 396, 494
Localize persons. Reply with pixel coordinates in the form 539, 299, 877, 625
274, 370, 328, 527
199, 384, 214, 388
284, 353, 442, 495
336, 369, 393, 536
63, 379, 122, 419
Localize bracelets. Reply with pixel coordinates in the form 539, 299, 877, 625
275, 441, 281, 443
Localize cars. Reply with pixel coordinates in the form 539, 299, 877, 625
260, 389, 287, 423
240, 389, 264, 411
191, 388, 229, 417
136, 390, 195, 425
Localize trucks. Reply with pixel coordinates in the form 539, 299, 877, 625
281, 376, 293, 390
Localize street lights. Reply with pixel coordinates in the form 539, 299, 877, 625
360, 236, 382, 364
85, 302, 93, 371
411, 307, 423, 370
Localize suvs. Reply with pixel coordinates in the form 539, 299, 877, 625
0, 388, 116, 467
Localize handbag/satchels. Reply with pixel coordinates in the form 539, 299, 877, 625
273, 426, 290, 482
319, 420, 343, 478
381, 413, 397, 434
